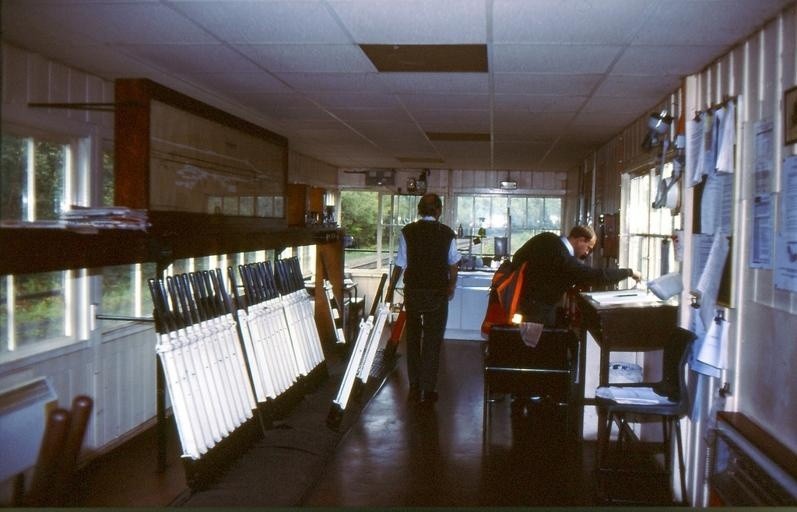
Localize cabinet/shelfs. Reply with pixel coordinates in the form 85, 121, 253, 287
444, 271, 496, 341
285, 183, 346, 338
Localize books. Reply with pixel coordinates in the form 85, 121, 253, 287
64, 205, 152, 235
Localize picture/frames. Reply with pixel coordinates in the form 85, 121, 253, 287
783, 85, 797, 148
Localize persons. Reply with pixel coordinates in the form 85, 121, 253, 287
384, 193, 463, 403
503, 224, 642, 327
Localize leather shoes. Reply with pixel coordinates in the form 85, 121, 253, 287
407, 384, 421, 403
424, 390, 440, 401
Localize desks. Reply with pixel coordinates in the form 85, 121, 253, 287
575, 289, 679, 497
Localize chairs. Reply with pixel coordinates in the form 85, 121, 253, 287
482, 326, 576, 466
596, 327, 698, 503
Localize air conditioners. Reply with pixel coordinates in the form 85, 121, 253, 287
0, 376, 62, 488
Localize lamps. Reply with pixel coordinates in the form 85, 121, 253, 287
500, 171, 518, 190
639, 110, 685, 211
